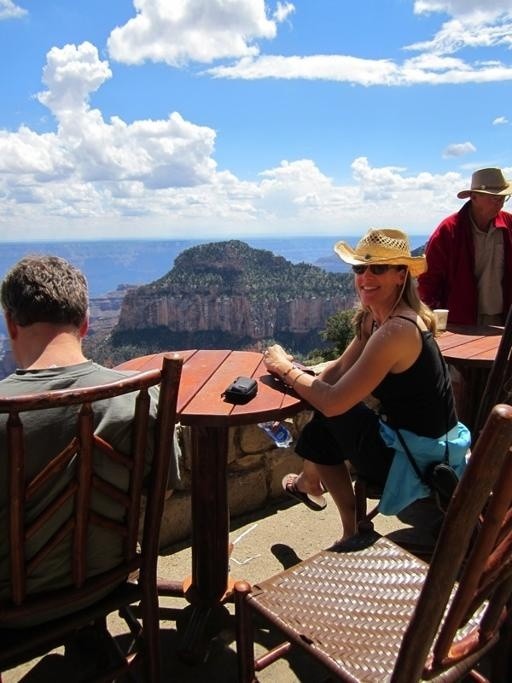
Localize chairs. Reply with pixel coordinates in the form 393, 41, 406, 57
0, 352, 184, 683
234, 403, 512, 682
349, 303, 511, 564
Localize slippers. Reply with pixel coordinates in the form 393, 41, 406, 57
281, 473, 327, 511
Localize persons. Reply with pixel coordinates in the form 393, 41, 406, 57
264, 229, 471, 545
417, 168, 512, 428
0, 256, 179, 649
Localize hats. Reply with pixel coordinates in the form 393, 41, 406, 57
334, 228, 429, 277
456, 167, 512, 199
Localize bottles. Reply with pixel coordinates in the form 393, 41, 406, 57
256, 419, 293, 448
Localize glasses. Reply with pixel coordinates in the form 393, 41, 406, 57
352, 264, 389, 275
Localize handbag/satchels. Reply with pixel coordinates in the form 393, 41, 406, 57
427, 463, 460, 503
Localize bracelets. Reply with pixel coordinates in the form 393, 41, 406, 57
293, 372, 305, 389
282, 366, 296, 381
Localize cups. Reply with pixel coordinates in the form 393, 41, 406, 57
433, 309, 449, 332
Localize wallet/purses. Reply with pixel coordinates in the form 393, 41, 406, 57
220, 376, 259, 405
